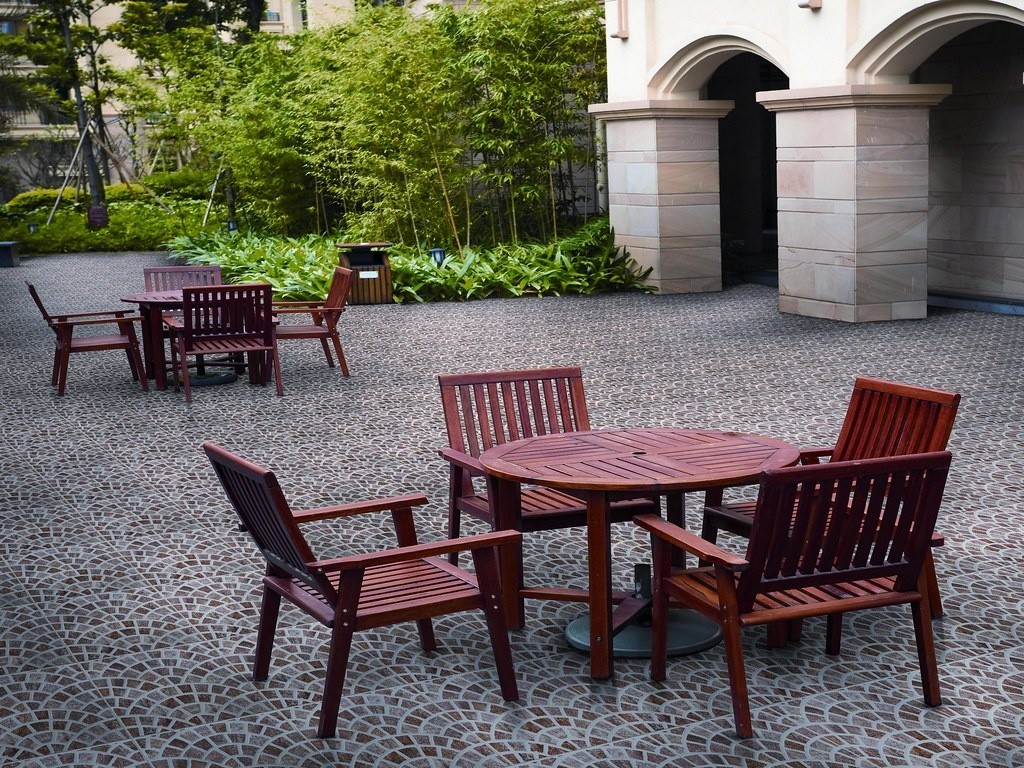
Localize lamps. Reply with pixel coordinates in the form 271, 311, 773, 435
227, 219, 238, 230
430, 247, 445, 266
28, 224, 38, 233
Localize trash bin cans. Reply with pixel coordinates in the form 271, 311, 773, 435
336, 241, 394, 304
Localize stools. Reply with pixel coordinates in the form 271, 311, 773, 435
0, 241, 20, 268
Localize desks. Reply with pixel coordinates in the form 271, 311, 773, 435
121, 289, 264, 390
479, 428, 800, 679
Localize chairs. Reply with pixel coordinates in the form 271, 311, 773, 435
144, 267, 355, 403
25, 279, 149, 395
635, 451, 952, 739
439, 367, 662, 588
700, 374, 962, 655
204, 442, 523, 738
